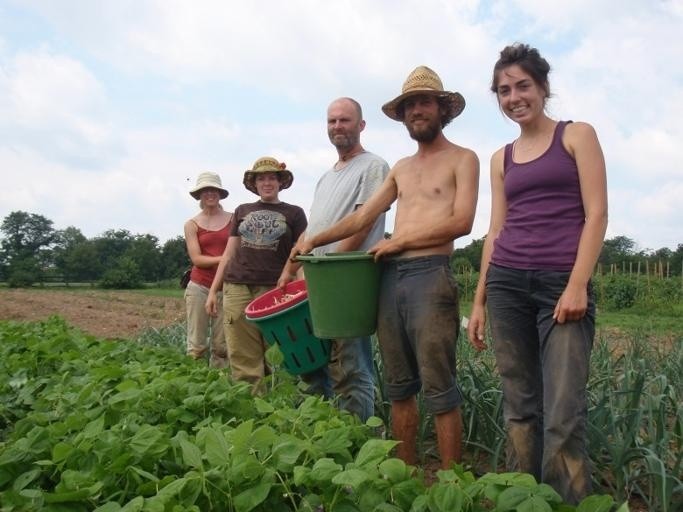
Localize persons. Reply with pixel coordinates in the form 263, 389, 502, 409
288, 65, 480, 471
468, 42, 608, 507
184, 172, 236, 372
276, 98, 391, 451
205, 157, 307, 399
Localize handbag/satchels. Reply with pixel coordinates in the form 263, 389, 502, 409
179, 271, 191, 289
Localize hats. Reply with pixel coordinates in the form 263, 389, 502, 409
243, 156, 294, 195
381, 65, 467, 126
189, 171, 229, 202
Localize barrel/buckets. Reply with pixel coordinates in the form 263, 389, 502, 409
292, 251, 384, 340
